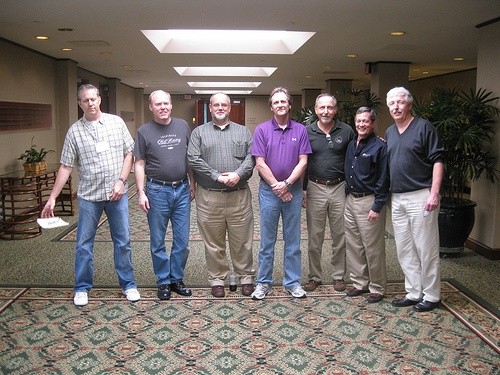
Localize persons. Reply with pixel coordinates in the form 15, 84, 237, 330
40, 84, 140, 306
250, 87, 312, 299
187, 93, 255, 299
343, 107, 391, 303
384, 87, 445, 312
302, 93, 387, 292
133, 90, 196, 300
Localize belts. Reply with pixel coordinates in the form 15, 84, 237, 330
147, 176, 187, 188
202, 185, 247, 192
350, 192, 374, 198
308, 175, 344, 186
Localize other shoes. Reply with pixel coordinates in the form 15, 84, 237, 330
368, 293, 383, 303
347, 287, 369, 296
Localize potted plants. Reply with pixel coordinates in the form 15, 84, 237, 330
408, 86, 500, 257
18, 136, 56, 172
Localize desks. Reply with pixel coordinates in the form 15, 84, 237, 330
0, 164, 75, 239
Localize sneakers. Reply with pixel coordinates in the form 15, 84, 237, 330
121, 288, 140, 301
252, 285, 269, 299
73, 291, 88, 306
286, 285, 307, 298
304, 280, 321, 291
333, 278, 346, 292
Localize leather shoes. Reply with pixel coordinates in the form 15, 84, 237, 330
158, 285, 170, 300
392, 297, 420, 307
414, 299, 441, 312
242, 284, 253, 296
212, 285, 225, 297
171, 281, 192, 296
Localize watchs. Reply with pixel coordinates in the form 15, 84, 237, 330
284, 180, 291, 188
119, 177, 127, 185
